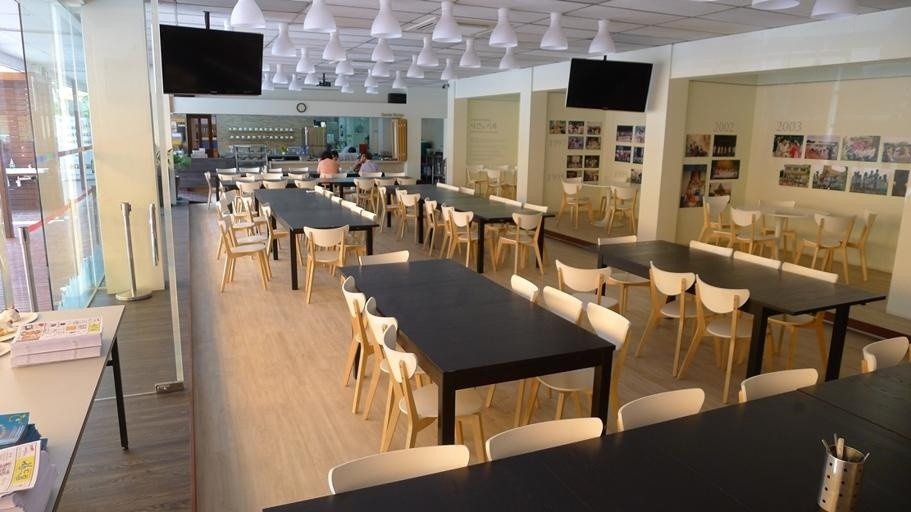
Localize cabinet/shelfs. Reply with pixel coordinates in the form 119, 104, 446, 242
228, 130, 296, 141
304, 125, 328, 147
391, 118, 409, 162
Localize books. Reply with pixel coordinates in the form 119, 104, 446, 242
9, 315, 107, 370
1, 411, 60, 512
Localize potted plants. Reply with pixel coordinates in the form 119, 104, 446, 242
156, 148, 193, 199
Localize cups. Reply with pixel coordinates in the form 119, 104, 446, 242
228, 126, 294, 132
229, 135, 294, 139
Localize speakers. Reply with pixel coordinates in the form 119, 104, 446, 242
387, 93, 406, 104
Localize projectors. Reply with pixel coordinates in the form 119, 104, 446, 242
315, 81, 331, 87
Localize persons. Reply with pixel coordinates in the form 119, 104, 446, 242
331, 152, 343, 172
352, 152, 368, 173
359, 152, 380, 177
348, 147, 357, 154
317, 150, 340, 188
778, 138, 911, 193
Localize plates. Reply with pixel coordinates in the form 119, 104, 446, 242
0, 310, 40, 360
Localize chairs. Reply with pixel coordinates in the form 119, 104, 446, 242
205, 165, 556, 304
634, 260, 714, 377
597, 234, 650, 312
485, 274, 632, 428
861, 336, 911, 373
328, 387, 706, 497
554, 259, 619, 310
676, 273, 771, 380
341, 276, 482, 465
739, 367, 819, 403
556, 175, 638, 235
698, 196, 877, 285
688, 239, 839, 369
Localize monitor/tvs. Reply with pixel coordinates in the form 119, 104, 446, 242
565, 57, 653, 112
159, 24, 264, 95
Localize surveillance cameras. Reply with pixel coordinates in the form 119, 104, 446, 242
442, 84, 449, 88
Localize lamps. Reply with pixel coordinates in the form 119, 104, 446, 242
809, 1, 859, 21
750, 0, 799, 10
224, 0, 616, 94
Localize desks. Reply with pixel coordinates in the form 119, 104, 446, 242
602, 240, 887, 381
0, 306, 129, 510
337, 258, 615, 446
